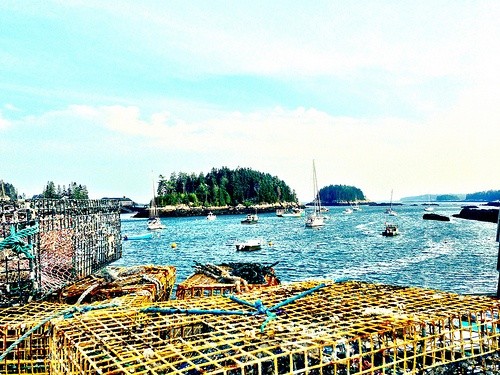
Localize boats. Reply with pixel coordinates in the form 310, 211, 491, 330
342, 208, 353, 214
276, 205, 304, 217
316, 206, 329, 213
235, 238, 266, 252
124, 232, 154, 240
206, 211, 217, 221
382, 224, 399, 237
241, 214, 259, 225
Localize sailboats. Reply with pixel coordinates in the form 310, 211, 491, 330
146, 169, 162, 230
387, 188, 397, 216
352, 194, 362, 211
305, 159, 325, 228
424, 194, 435, 212
